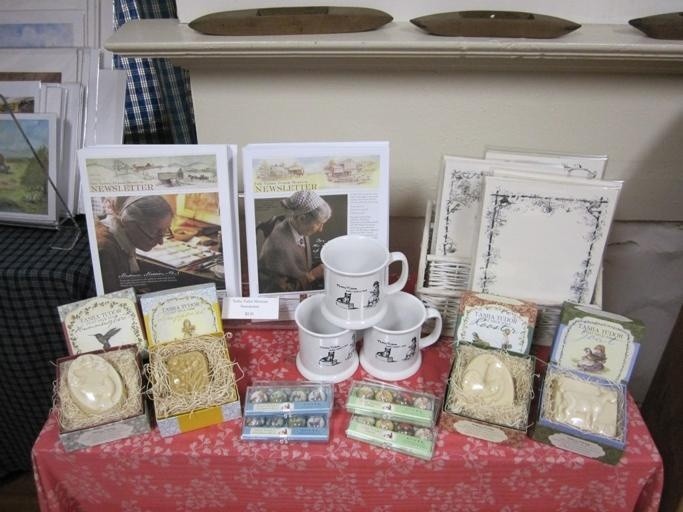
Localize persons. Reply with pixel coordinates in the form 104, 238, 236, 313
95, 194, 174, 295
369, 281, 380, 298
258, 189, 332, 294
409, 337, 416, 351
593, 344, 608, 364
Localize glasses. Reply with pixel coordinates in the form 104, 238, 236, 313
136, 223, 174, 243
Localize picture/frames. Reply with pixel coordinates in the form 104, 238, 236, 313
0, 1, 132, 230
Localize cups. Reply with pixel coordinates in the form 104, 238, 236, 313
293, 293, 362, 388
317, 235, 409, 330
359, 290, 444, 383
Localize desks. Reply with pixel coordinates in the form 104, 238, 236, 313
28, 269, 665, 510
2, 212, 99, 479
